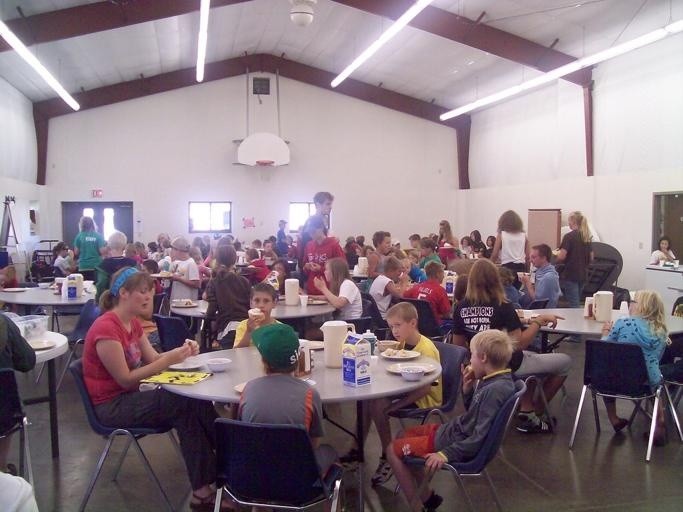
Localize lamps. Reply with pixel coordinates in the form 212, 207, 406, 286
0, 22, 80, 111
331, 0, 433, 87
195, 0, 211, 82
438, 19, 683, 122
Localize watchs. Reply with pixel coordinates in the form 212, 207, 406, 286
602, 326, 612, 330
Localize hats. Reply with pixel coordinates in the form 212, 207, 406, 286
170, 237, 191, 251
302, 215, 323, 233
250, 322, 301, 368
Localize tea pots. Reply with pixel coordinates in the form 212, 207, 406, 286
592, 291, 613, 322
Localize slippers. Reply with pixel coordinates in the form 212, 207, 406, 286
642, 430, 667, 447
613, 418, 629, 433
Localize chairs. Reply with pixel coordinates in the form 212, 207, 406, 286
359, 292, 392, 339
397, 297, 453, 344
342, 316, 372, 334
213, 417, 344, 512
35, 299, 101, 391
68, 358, 183, 512
395, 378, 529, 511
388, 341, 468, 432
0, 368, 34, 487
153, 314, 209, 354
50, 265, 66, 277
569, 339, 683, 463
624, 295, 682, 427
153, 292, 166, 314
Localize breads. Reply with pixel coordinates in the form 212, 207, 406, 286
248, 308, 265, 323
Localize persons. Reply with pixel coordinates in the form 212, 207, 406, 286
403, 259, 452, 334
276, 219, 288, 255
0, 314, 37, 476
297, 191, 334, 283
205, 244, 252, 350
286, 234, 298, 257
231, 282, 284, 348
438, 219, 460, 269
649, 234, 677, 267
235, 241, 245, 253
295, 225, 304, 260
262, 240, 279, 261
147, 241, 159, 259
519, 242, 562, 308
312, 257, 363, 329
270, 258, 293, 294
410, 232, 422, 251
391, 240, 406, 256
497, 264, 521, 307
470, 229, 487, 255
269, 236, 277, 251
364, 230, 408, 294
190, 237, 208, 260
490, 210, 532, 288
73, 216, 110, 279
385, 328, 516, 512
416, 237, 445, 270
598, 288, 670, 447
486, 235, 496, 258
339, 300, 445, 486
165, 237, 201, 304
235, 322, 349, 511
369, 254, 417, 320
52, 242, 74, 277
451, 256, 574, 433
344, 234, 374, 261
124, 243, 143, 263
94, 231, 138, 301
244, 247, 271, 281
202, 237, 234, 266
449, 272, 469, 316
300, 216, 347, 299
159, 233, 171, 253
460, 237, 474, 256
80, 262, 249, 511
252, 239, 263, 249
553, 210, 596, 344
134, 240, 149, 260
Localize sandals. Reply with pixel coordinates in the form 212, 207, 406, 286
189, 491, 238, 512
416, 489, 444, 512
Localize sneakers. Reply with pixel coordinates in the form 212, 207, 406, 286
370, 456, 394, 485
535, 414, 557, 429
334, 447, 360, 473
516, 409, 553, 434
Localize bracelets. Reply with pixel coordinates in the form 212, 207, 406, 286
532, 318, 542, 329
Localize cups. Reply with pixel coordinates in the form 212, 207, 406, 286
619, 300, 630, 316
672, 259, 679, 268
300, 296, 308, 309
659, 259, 665, 267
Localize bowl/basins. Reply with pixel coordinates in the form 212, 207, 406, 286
376, 341, 400, 353
38, 283, 50, 289
172, 299, 187, 304
401, 368, 424, 381
205, 358, 232, 372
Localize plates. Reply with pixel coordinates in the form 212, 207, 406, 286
2, 288, 31, 292
148, 273, 174, 278
380, 349, 421, 360
307, 300, 327, 305
172, 303, 199, 308
440, 247, 457, 250
386, 363, 437, 374
234, 378, 317, 394
27, 341, 57, 349
307, 341, 324, 350
167, 361, 203, 370
